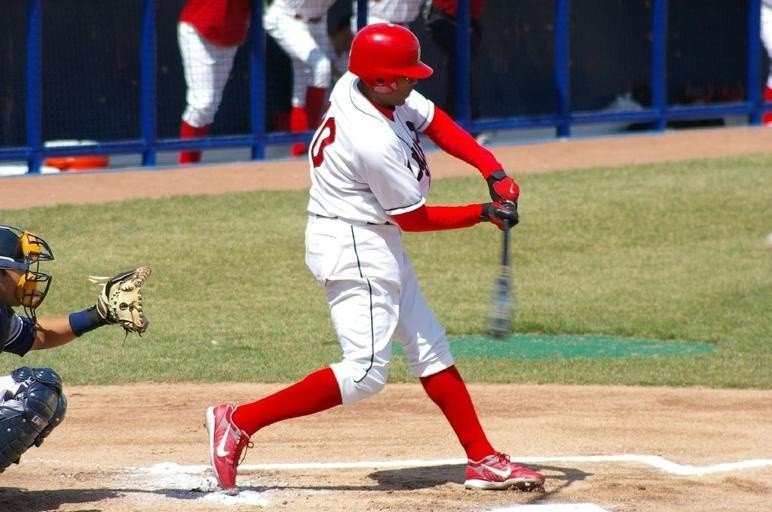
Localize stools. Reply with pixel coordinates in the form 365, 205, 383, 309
294, 14, 324, 22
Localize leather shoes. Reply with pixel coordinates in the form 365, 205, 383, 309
483, 206, 520, 229
485, 169, 520, 204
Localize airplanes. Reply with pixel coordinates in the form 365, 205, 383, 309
97, 264, 152, 346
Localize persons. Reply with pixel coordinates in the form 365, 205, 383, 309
262, 1, 338, 156
204, 23, 544, 490
757, 1, 771, 125
1, 226, 150, 479
175, 0, 268, 165
426, 1, 486, 121
346, 1, 448, 114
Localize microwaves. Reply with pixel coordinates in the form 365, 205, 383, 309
485, 218, 514, 341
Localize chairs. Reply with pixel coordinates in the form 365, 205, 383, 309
206, 402, 250, 494
464, 452, 544, 491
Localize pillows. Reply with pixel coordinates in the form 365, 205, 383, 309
348, 23, 432, 93
0, 225, 54, 324
